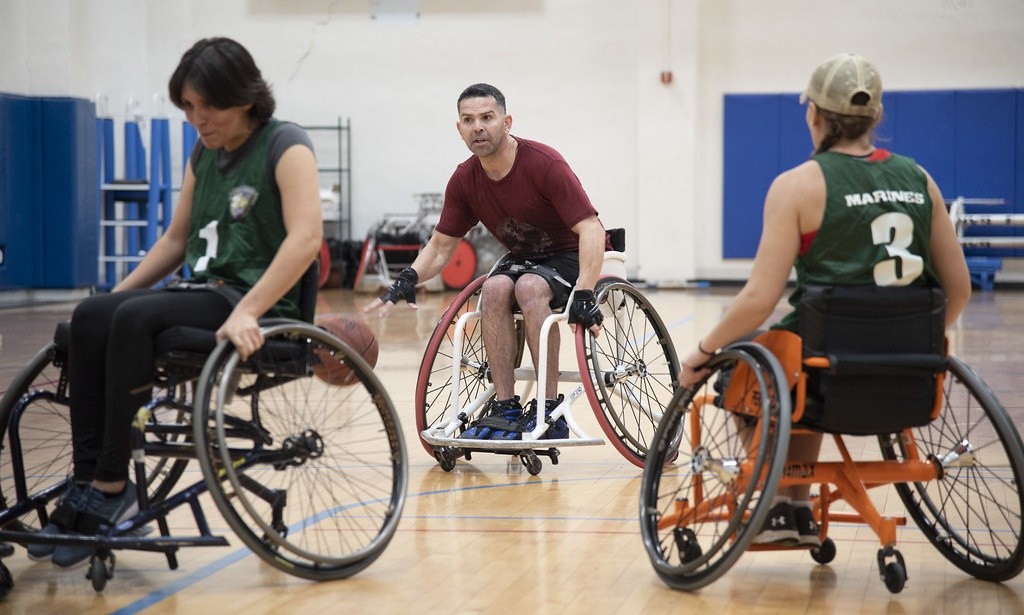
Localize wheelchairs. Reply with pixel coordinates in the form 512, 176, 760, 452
0, 254, 411, 591
414, 251, 687, 475
639, 274, 1023, 592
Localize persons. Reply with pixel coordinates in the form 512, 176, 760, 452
26, 35, 324, 573
679, 51, 973, 548
364, 83, 606, 443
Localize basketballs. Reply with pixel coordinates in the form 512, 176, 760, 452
307, 316, 380, 388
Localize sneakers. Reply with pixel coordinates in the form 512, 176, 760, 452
51, 480, 141, 570
793, 506, 822, 548
457, 395, 524, 440
751, 503, 800, 546
26, 484, 90, 561
491, 394, 569, 440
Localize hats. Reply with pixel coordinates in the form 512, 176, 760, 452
799, 53, 883, 118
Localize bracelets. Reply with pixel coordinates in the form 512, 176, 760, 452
698, 340, 716, 355
